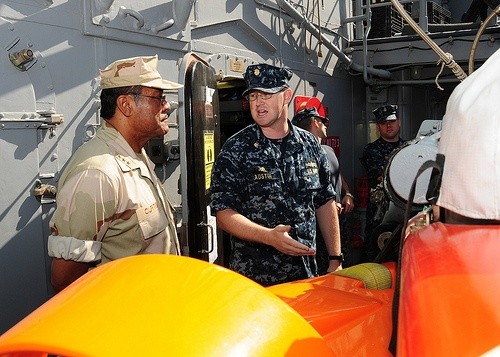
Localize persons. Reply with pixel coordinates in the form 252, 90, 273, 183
360, 104, 406, 263
210, 63, 345, 288
436, 47, 500, 225
291, 106, 355, 278
48, 54, 180, 293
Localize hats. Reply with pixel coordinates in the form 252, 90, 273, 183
100, 54, 184, 90
291, 106, 329, 126
242, 63, 293, 96
371, 105, 399, 124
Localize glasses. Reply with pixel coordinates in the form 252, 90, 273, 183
114, 92, 166, 105
245, 91, 282, 101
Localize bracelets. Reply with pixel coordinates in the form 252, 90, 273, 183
346, 192, 354, 200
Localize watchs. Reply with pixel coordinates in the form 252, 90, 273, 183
328, 252, 344, 262
